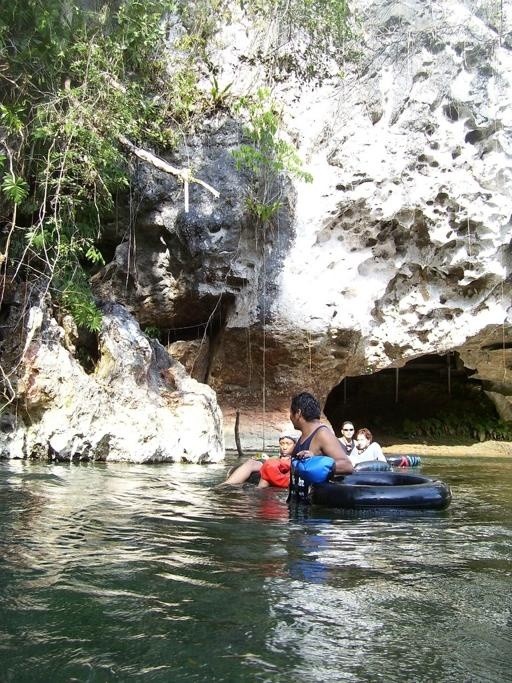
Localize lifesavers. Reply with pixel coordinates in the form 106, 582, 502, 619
227, 458, 266, 484
353, 461, 392, 471
310, 469, 451, 510
385, 454, 421, 466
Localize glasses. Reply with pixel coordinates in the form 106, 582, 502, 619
343, 428, 355, 432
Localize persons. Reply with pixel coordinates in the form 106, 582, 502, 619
334, 420, 360, 461
349, 426, 388, 467
218, 434, 299, 489
288, 390, 355, 504
